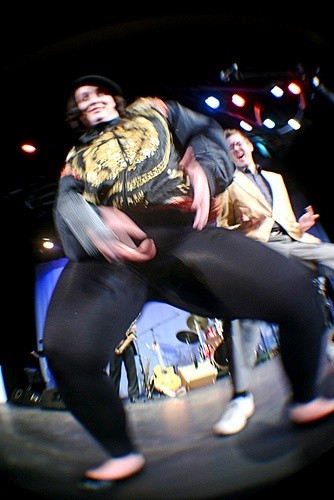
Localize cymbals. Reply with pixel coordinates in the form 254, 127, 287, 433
187, 314, 208, 330
176, 330, 198, 343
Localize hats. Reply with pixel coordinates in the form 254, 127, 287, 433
70, 73, 122, 97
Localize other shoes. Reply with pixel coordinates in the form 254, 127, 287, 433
129, 396, 146, 404
214, 392, 256, 436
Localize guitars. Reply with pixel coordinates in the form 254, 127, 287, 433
147, 328, 181, 393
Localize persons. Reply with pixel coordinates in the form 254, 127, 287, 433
210, 128, 333, 436
53, 75, 334, 494
110, 323, 138, 403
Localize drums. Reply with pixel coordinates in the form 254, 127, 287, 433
213, 341, 257, 370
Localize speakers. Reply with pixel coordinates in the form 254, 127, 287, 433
11, 388, 40, 406
40, 389, 69, 410
176, 362, 218, 391
